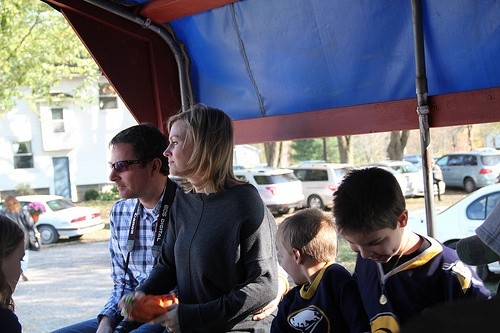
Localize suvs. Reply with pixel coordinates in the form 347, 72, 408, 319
233, 154, 446, 215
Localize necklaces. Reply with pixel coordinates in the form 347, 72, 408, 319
374, 235, 410, 305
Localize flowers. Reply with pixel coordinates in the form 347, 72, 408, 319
27, 201, 46, 224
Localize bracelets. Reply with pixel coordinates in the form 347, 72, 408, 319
279, 273, 289, 295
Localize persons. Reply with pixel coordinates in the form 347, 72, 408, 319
50, 122, 288, 333
118, 102, 279, 333
0, 195, 42, 333
270, 167, 500, 333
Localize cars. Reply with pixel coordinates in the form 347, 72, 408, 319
0, 194, 105, 244
404, 183, 500, 283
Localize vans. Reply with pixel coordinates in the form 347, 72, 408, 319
434, 147, 500, 193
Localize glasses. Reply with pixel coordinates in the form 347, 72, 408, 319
108, 158, 151, 173
